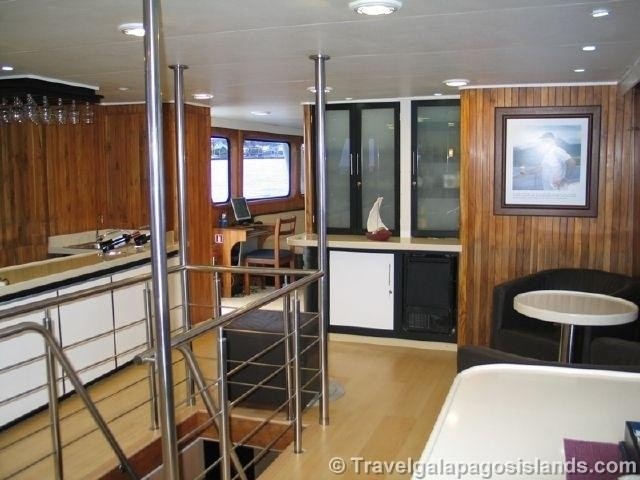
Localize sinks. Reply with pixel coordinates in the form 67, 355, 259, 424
63, 243, 146, 255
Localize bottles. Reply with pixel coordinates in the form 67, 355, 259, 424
101, 231, 141, 252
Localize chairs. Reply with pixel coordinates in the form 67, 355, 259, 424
244, 214, 296, 295
458, 268, 640, 375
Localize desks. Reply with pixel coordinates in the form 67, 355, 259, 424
212, 223, 277, 298
410, 363, 639, 480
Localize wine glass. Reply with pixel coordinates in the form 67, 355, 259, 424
0, 94, 94, 126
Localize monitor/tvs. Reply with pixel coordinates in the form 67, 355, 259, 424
231, 197, 254, 224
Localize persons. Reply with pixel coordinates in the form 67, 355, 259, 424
520, 132, 575, 190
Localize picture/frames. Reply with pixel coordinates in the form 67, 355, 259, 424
493, 105, 599, 218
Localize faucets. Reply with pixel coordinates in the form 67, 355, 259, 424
96, 214, 104, 242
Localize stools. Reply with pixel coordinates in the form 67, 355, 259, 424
222, 310, 319, 412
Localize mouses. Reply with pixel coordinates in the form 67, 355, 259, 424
255, 221, 262, 224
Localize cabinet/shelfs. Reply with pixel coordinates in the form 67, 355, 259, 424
327, 246, 403, 338
313, 102, 399, 236
411, 100, 460, 238
